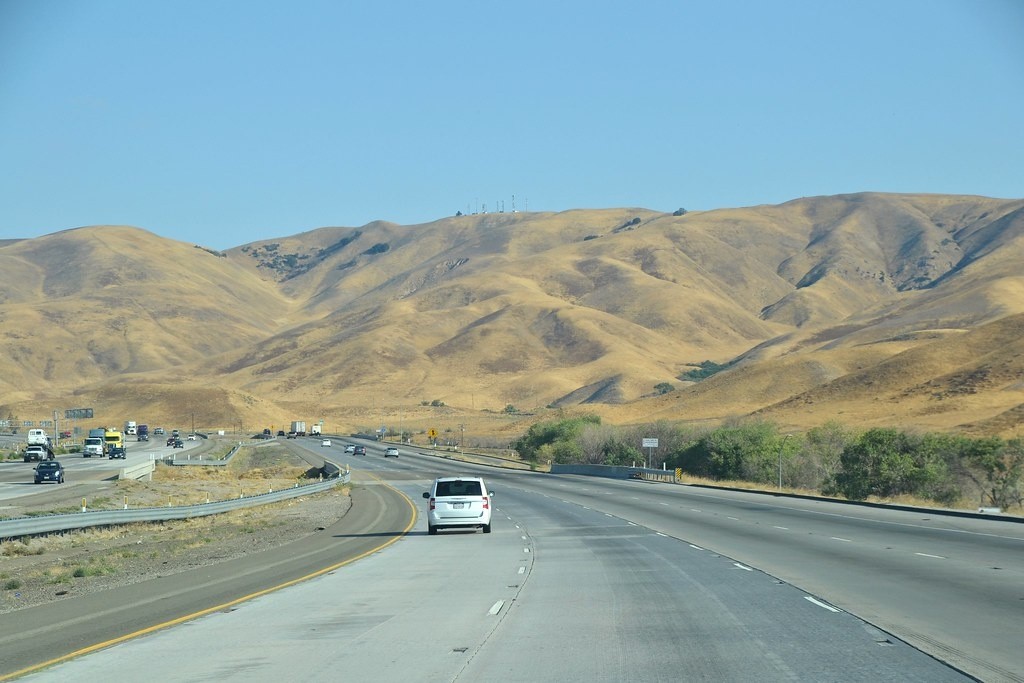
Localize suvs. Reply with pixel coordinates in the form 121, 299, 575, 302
423, 476, 496, 534
21, 444, 49, 463
81, 437, 104, 457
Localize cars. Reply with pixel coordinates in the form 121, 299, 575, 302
167, 437, 176, 446
321, 439, 331, 447
352, 445, 367, 456
154, 427, 164, 434
286, 431, 296, 440
33, 461, 65, 485
383, 447, 399, 458
186, 434, 196, 441
276, 431, 284, 436
172, 440, 184, 448
172, 430, 180, 440
108, 447, 127, 459
263, 429, 270, 435
343, 444, 356, 453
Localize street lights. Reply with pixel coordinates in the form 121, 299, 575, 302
777, 434, 794, 491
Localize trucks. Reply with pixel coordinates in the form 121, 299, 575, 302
309, 425, 322, 436
103, 431, 125, 454
290, 421, 306, 437
88, 429, 106, 447
136, 425, 149, 441
60, 431, 71, 438
124, 420, 137, 435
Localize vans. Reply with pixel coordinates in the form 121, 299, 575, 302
27, 428, 48, 446
